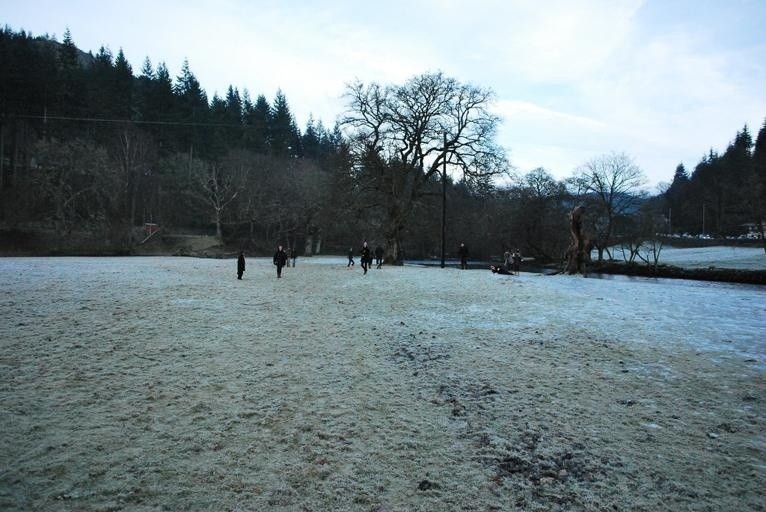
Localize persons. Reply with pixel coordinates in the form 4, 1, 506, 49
460, 242, 468, 269
237, 251, 245, 279
347, 247, 354, 266
489, 248, 523, 275
273, 245, 296, 278
360, 239, 384, 274
